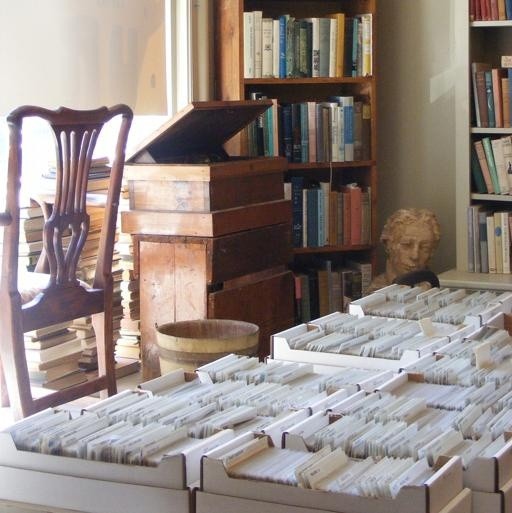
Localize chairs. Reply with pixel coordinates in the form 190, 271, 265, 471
0, 101, 133, 421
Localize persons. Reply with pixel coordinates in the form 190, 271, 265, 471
366, 208, 441, 294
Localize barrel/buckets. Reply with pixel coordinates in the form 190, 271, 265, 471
150, 317, 260, 380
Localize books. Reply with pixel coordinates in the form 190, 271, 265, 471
471, 62, 512, 128
282, 182, 372, 247
468, 0, 512, 21
18, 152, 140, 391
244, 11, 372, 77
471, 135, 512, 195
467, 205, 512, 275
247, 93, 370, 162
294, 259, 372, 324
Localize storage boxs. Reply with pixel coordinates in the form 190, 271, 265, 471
123, 94, 291, 244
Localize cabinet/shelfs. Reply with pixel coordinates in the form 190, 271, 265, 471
451, 0, 512, 277
218, 0, 378, 323
